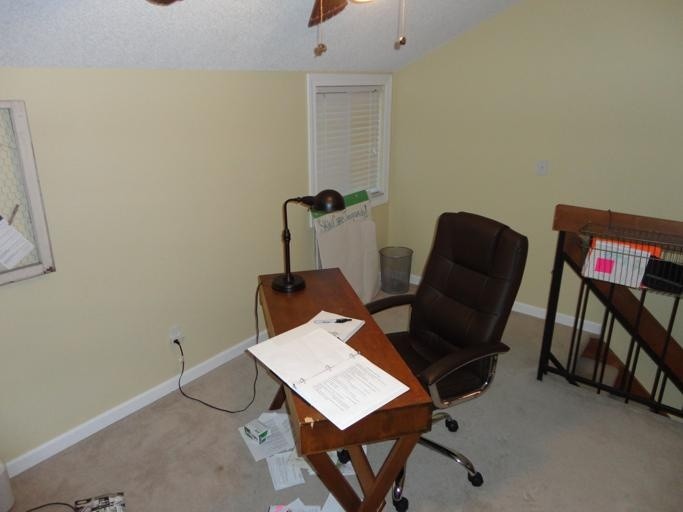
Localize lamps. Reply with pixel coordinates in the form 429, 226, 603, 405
271, 190, 345, 292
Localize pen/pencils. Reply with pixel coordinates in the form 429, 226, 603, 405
7, 205, 19, 226
315, 318, 353, 323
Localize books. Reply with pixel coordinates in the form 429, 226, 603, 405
581, 236, 664, 289
247, 320, 411, 431
310, 310, 367, 341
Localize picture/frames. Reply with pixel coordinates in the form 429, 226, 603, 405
1, 101, 57, 288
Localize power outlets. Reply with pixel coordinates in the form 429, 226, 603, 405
170, 334, 183, 347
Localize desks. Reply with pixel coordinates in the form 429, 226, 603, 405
257, 266, 435, 510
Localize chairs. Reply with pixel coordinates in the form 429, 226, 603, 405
336, 210, 533, 510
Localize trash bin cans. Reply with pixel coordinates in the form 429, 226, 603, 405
379, 245, 414, 294
